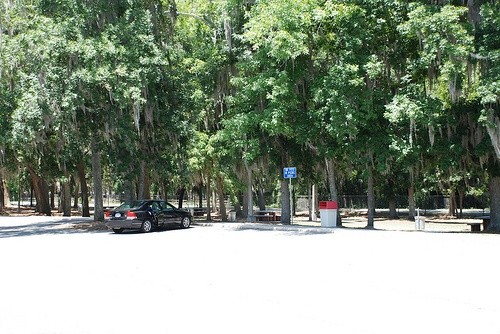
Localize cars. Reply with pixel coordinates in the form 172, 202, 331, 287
105, 200, 194, 233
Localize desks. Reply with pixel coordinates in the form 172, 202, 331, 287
185, 208, 203, 216
255, 210, 281, 221
474, 216, 490, 230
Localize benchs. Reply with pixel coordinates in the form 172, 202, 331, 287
194, 211, 204, 213
253, 214, 273, 221
467, 223, 483, 231
